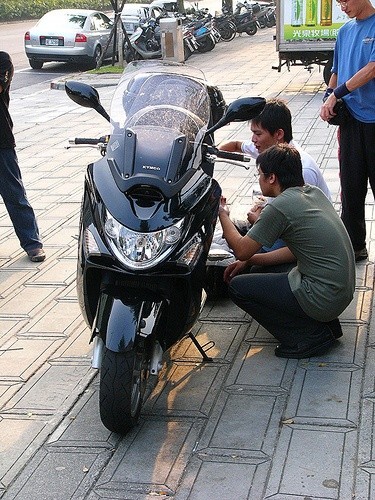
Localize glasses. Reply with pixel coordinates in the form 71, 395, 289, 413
336, 0, 349, 8
254, 173, 268, 178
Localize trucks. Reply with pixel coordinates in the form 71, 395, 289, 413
272, 0, 375, 87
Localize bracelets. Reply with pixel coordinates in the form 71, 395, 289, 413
323, 87, 334, 102
333, 81, 352, 99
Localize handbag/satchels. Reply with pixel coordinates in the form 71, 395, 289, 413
323, 97, 346, 125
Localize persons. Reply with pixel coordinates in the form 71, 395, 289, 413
215, 99, 332, 252
321, 0, 375, 261
219, 142, 357, 360
0, 51, 46, 261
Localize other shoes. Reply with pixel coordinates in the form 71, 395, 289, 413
27, 248, 46, 261
274, 325, 335, 359
328, 318, 343, 339
354, 247, 368, 262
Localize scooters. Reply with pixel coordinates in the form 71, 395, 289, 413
123, 0, 277, 61
61, 59, 267, 436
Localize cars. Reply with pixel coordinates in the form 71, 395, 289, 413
24, 8, 127, 69
109, 0, 198, 35
